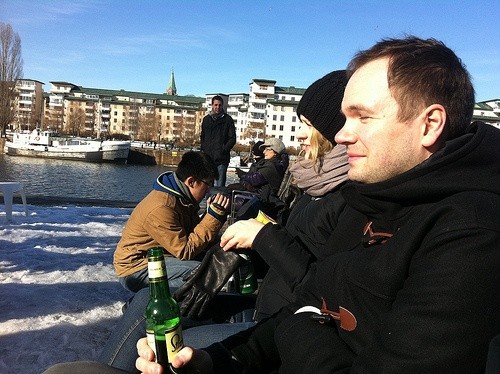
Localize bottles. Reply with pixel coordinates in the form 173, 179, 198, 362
232, 249, 259, 295
146, 246, 185, 374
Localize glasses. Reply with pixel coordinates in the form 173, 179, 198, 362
196, 178, 213, 189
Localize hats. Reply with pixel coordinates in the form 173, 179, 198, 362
296, 70, 354, 147
259, 138, 285, 154
251, 141, 265, 156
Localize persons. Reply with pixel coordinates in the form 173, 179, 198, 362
42, 37, 500, 374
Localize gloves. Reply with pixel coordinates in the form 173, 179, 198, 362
173, 242, 242, 320
235, 167, 246, 178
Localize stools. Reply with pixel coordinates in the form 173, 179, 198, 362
0, 181, 28, 220
